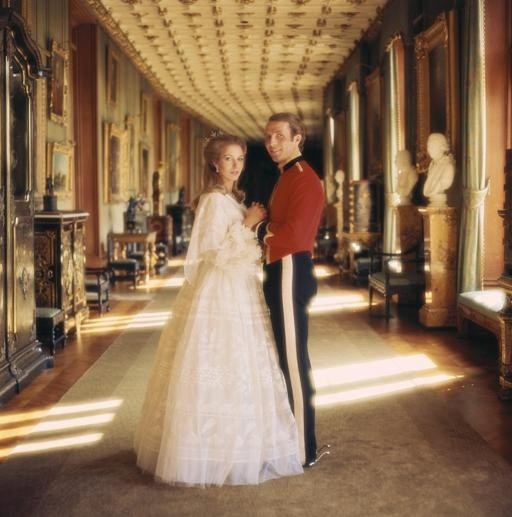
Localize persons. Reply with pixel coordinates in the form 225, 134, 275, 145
326, 169, 344, 201
133, 134, 305, 488
253, 113, 325, 468
395, 150, 419, 195
423, 133, 455, 197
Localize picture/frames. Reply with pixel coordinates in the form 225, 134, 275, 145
414, 11, 461, 175
47, 142, 74, 197
47, 39, 71, 125
103, 46, 180, 203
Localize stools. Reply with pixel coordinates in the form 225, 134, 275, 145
456, 287, 512, 400
35, 307, 68, 356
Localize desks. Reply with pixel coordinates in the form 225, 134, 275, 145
113, 232, 156, 286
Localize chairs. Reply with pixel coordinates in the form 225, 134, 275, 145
368, 249, 425, 321
107, 232, 140, 290
85, 267, 111, 315
